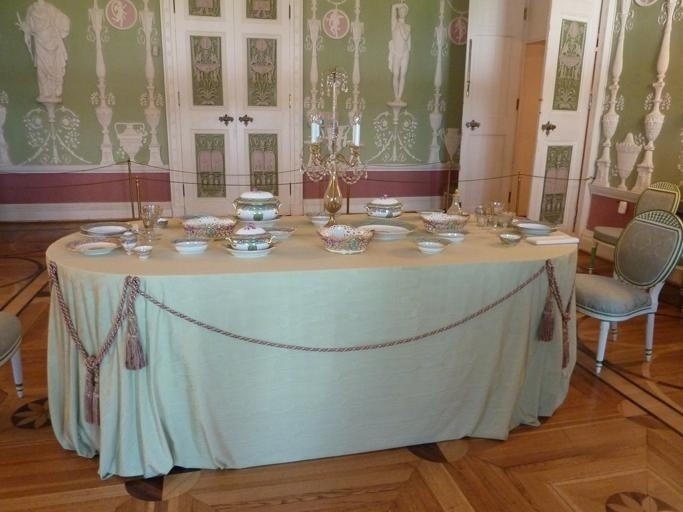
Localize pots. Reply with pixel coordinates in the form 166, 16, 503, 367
363, 194, 405, 220
225, 223, 277, 250
232, 187, 282, 225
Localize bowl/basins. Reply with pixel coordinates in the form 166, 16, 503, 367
132, 245, 152, 259
156, 217, 168, 228
498, 231, 523, 248
117, 235, 138, 256
419, 211, 470, 234
309, 216, 331, 227
180, 214, 239, 240
225, 246, 275, 258
316, 224, 374, 254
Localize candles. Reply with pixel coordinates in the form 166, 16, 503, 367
352, 115, 361, 146
311, 114, 321, 144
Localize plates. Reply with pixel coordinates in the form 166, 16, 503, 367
512, 220, 557, 237
355, 219, 416, 241
66, 237, 121, 256
171, 239, 209, 256
435, 230, 470, 243
80, 221, 132, 238
263, 226, 296, 240
414, 238, 453, 256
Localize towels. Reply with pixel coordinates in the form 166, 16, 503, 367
527, 234, 580, 245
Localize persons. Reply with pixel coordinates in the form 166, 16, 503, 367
16, 2, 69, 100
391, 2, 411, 103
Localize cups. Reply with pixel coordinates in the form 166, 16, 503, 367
498, 213, 511, 233
492, 201, 502, 216
141, 212, 160, 246
143, 204, 162, 237
475, 205, 487, 226
505, 211, 516, 228
486, 216, 497, 233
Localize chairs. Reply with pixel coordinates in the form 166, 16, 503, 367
571, 211, 677, 372
587, 181, 681, 272
1, 310, 24, 400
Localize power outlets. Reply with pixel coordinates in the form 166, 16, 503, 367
618, 202, 628, 215
